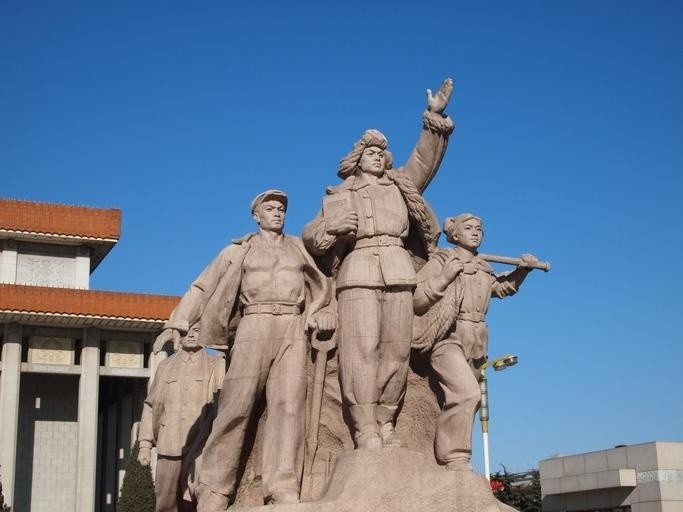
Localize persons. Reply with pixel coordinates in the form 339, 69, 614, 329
299, 76, 454, 451
134, 316, 224, 510
411, 211, 540, 472
150, 189, 339, 511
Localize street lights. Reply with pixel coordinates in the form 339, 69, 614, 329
477, 353, 520, 482
